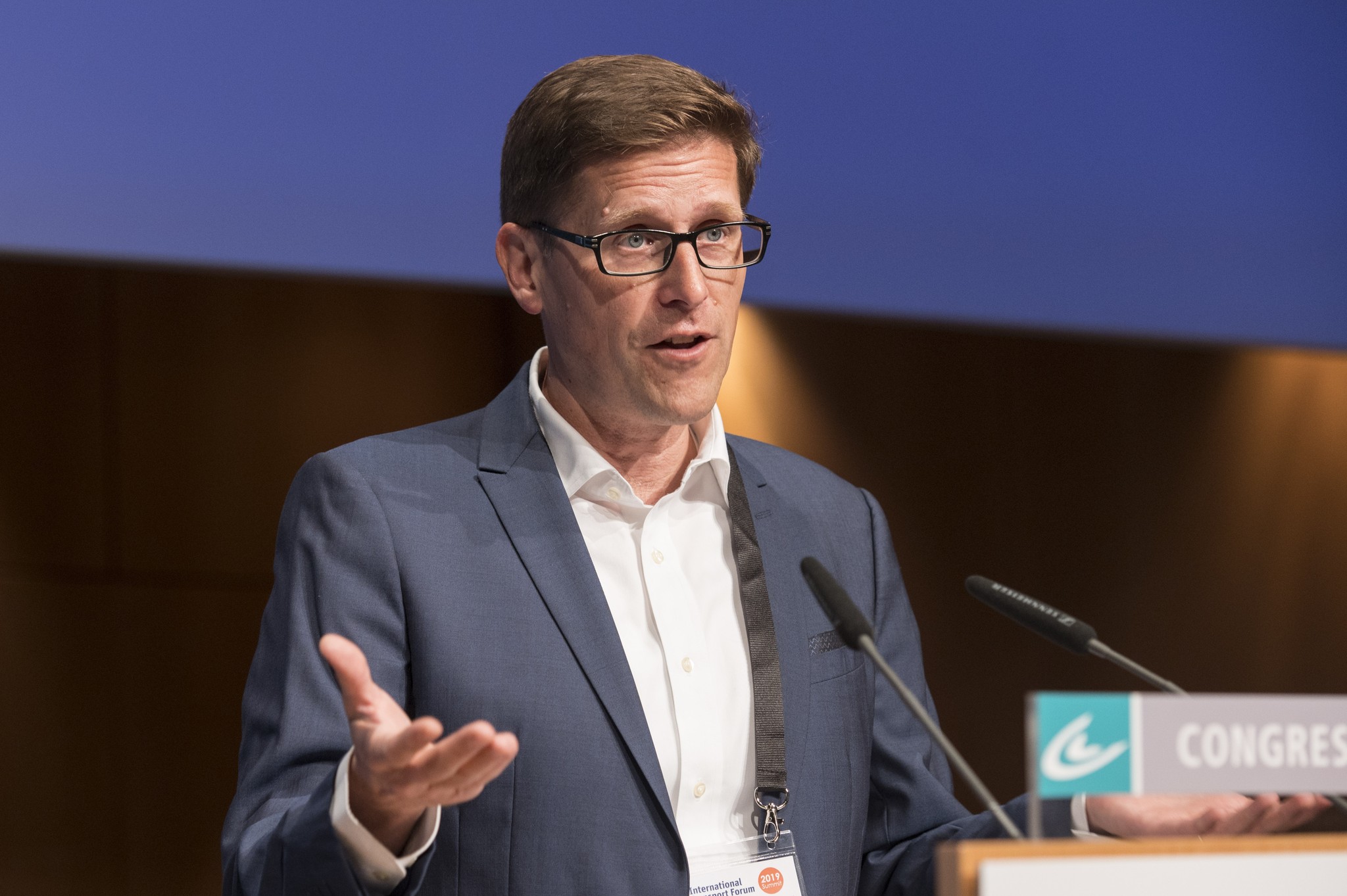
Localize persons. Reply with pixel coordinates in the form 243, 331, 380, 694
219, 53, 1331, 896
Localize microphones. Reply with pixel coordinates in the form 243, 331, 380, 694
963, 577, 1189, 691
802, 558, 1026, 837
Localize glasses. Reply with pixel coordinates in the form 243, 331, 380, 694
526, 213, 772, 276
